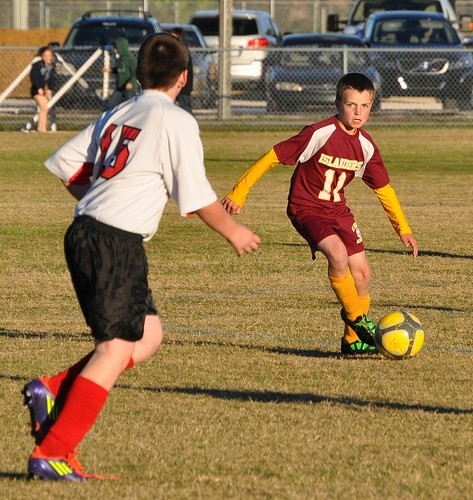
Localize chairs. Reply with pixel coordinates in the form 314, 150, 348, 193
290, 52, 308, 65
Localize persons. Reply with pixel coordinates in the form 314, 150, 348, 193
30, 46, 55, 133
102, 38, 138, 110
20, 32, 261, 481
171, 28, 193, 114
221, 73, 419, 353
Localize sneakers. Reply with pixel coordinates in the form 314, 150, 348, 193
341, 308, 377, 345
22, 381, 60, 447
27, 446, 119, 484
340, 337, 381, 356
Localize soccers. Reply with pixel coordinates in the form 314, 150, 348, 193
374, 310, 424, 360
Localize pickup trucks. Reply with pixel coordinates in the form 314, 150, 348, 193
46, 10, 215, 110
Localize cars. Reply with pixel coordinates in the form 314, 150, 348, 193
262, 33, 382, 112
324, 0, 473, 109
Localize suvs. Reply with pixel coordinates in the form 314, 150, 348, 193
186, 10, 293, 89
